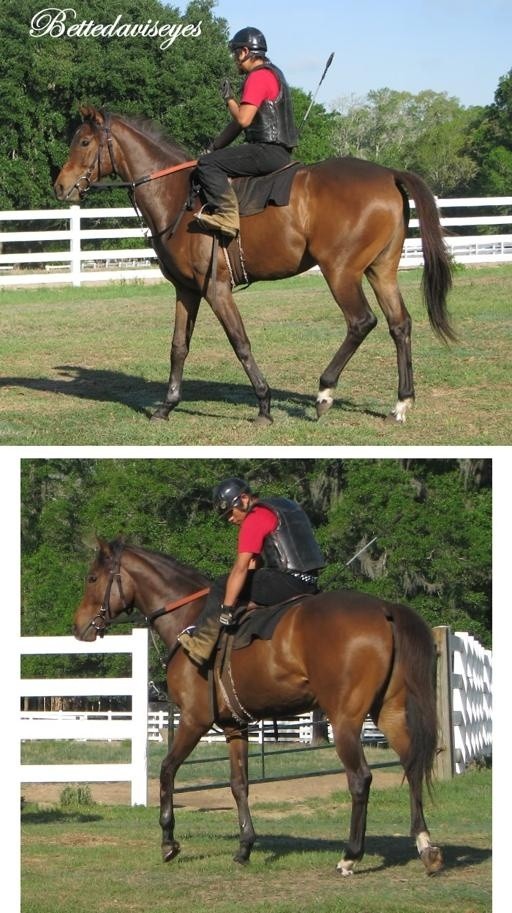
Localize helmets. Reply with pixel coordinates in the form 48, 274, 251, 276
210, 479, 249, 519
228, 27, 266, 52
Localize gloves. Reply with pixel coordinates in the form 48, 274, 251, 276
221, 78, 234, 100
219, 604, 236, 626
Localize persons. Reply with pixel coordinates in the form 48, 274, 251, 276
175, 476, 329, 669
187, 24, 303, 236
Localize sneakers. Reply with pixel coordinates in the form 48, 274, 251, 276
194, 212, 237, 237
176, 634, 205, 665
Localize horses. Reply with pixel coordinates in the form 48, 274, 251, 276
53, 105, 466, 427
71, 531, 445, 877
